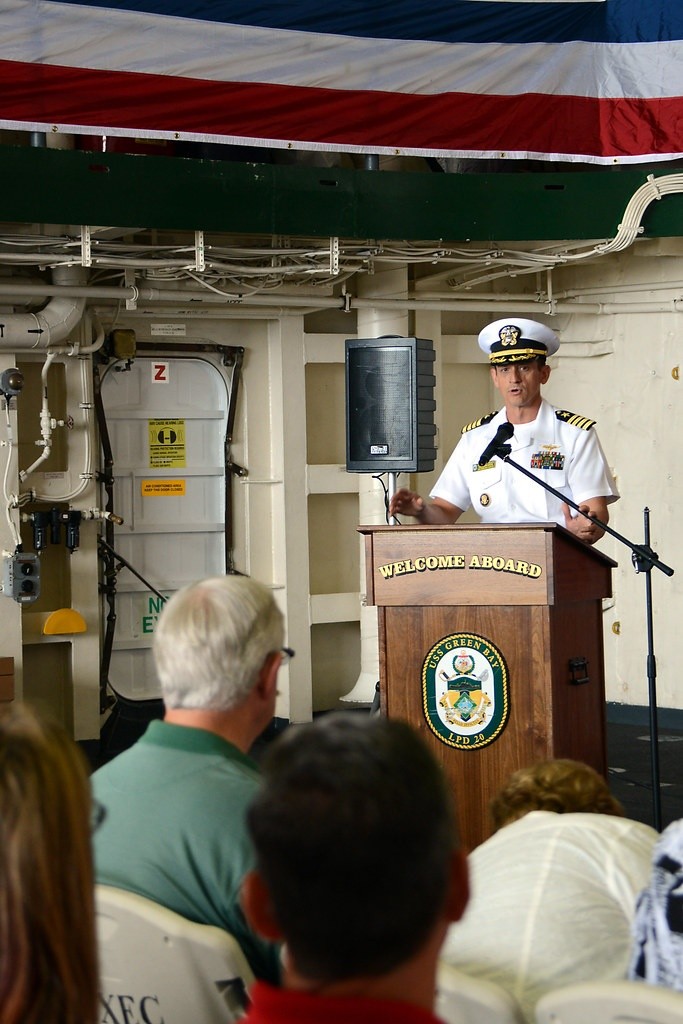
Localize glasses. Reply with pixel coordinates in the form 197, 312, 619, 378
279, 648, 291, 665
92, 799, 102, 835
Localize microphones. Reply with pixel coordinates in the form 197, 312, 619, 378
479, 422, 515, 466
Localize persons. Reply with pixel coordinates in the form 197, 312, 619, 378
226, 711, 471, 1022
623, 812, 683, 996
75, 570, 313, 988
0, 695, 109, 1024
430, 754, 661, 1021
379, 316, 620, 544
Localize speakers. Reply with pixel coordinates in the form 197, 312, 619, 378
343, 336, 438, 473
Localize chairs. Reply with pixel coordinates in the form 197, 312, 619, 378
91, 882, 257, 1024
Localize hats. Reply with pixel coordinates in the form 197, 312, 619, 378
477, 318, 560, 366
